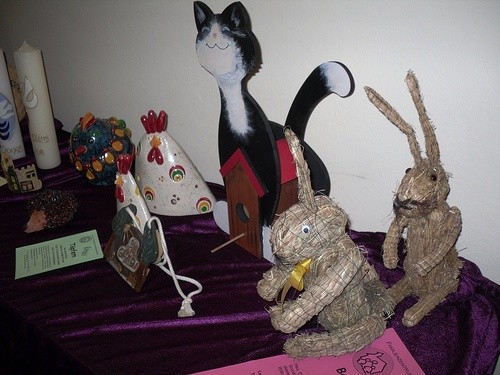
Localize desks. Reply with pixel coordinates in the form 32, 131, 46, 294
0, 118, 500, 375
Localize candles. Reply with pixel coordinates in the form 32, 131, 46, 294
14, 40, 62, 170
